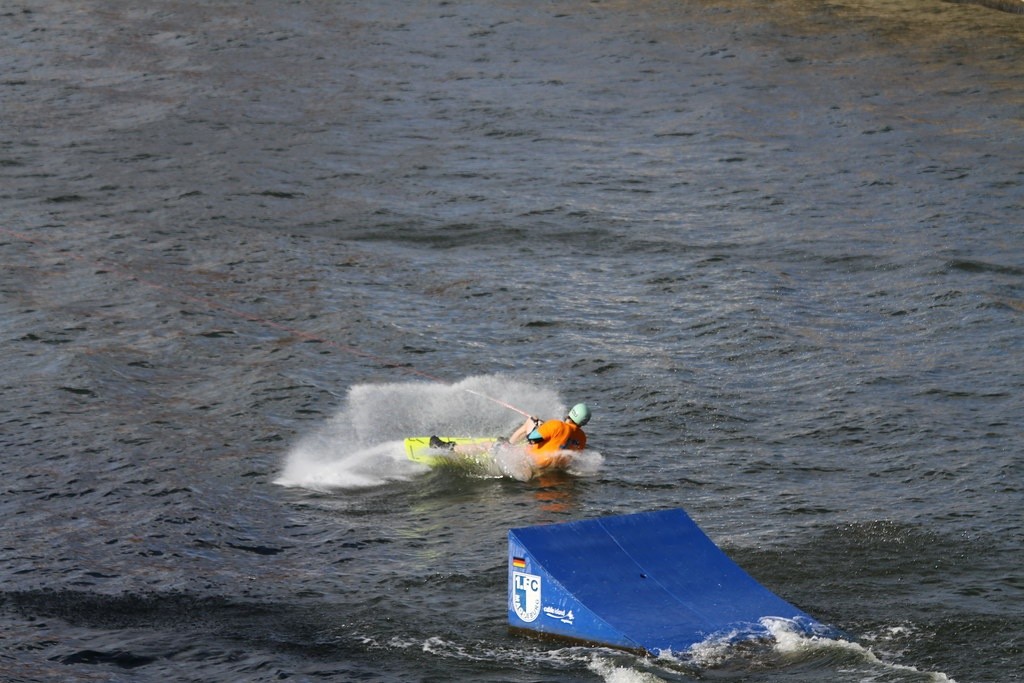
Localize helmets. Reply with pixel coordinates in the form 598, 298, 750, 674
568, 403, 591, 426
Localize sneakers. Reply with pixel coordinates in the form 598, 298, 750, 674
430, 435, 455, 461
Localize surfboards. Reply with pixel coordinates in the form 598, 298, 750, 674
403, 436, 535, 467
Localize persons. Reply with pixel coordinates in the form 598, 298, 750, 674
430, 402, 592, 478
